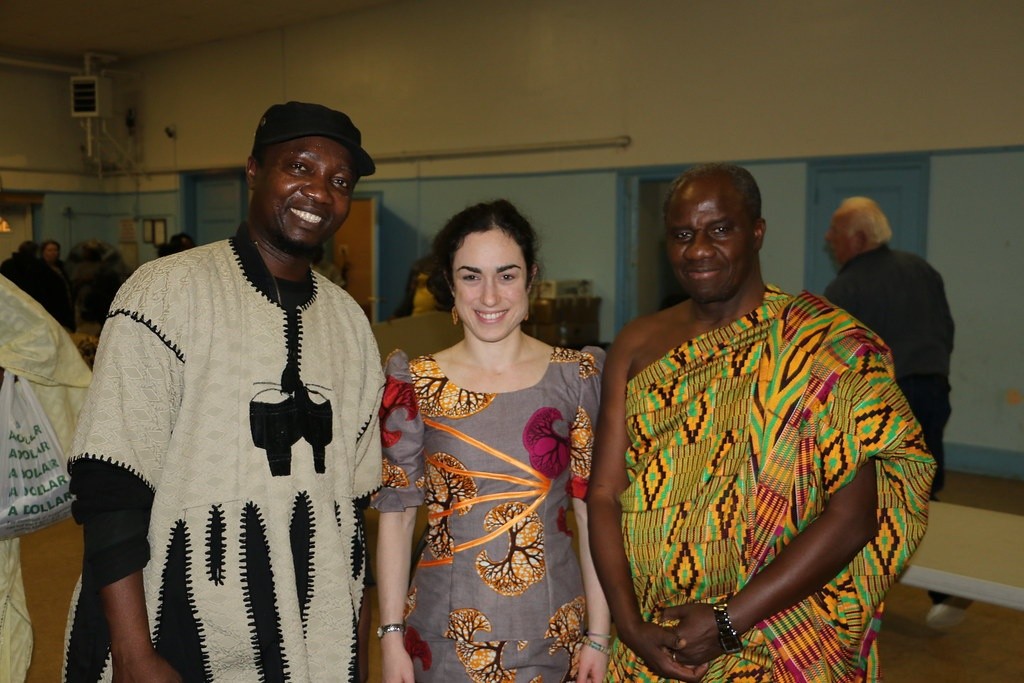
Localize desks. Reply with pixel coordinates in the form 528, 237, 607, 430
893, 498, 1023, 610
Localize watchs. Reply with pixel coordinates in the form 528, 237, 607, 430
375, 623, 407, 639
714, 598, 744, 654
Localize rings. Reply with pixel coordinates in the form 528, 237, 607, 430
670, 649, 679, 662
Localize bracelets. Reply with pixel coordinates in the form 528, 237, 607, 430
580, 631, 617, 656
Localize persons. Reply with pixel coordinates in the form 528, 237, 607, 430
0, 232, 203, 332
61, 101, 384, 683
823, 196, 976, 628
584, 165, 937, 683
377, 198, 612, 679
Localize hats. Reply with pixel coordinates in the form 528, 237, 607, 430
254, 101, 376, 176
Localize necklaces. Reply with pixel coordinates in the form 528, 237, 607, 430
251, 235, 283, 306
